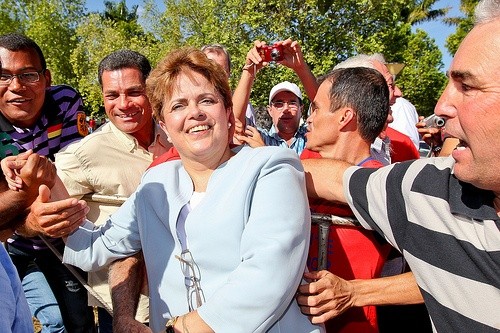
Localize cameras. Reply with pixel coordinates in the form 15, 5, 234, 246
421, 114, 446, 130
257, 44, 284, 62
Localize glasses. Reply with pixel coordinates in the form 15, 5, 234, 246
271, 101, 300, 108
0, 69, 45, 84
175, 249, 205, 311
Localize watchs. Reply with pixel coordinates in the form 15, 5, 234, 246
165, 315, 179, 333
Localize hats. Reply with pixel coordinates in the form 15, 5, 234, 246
269, 81, 303, 106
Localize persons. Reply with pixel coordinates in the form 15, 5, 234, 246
0, 0, 500, 333
